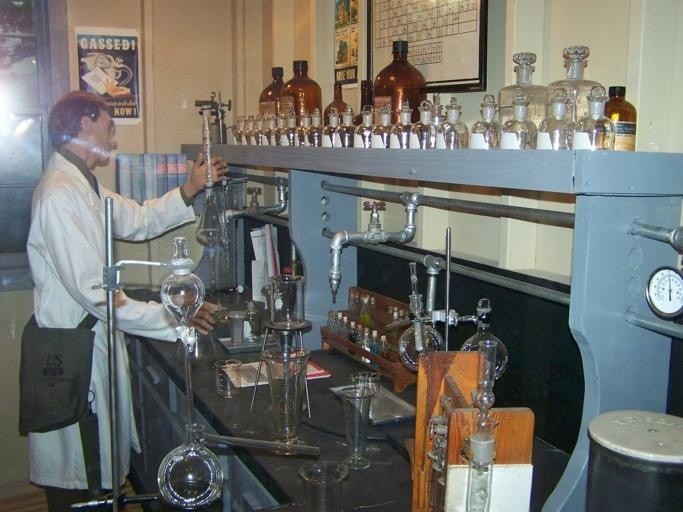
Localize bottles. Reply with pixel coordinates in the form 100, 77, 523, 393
572, 89, 615, 151
278, 61, 323, 147
380, 336, 388, 368
326, 311, 334, 328
262, 113, 279, 145
471, 96, 501, 148
370, 331, 381, 366
597, 83, 637, 154
241, 119, 254, 145
373, 41, 427, 125
354, 105, 377, 147
270, 113, 287, 145
390, 102, 416, 149
359, 298, 373, 324
387, 305, 409, 318
537, 88, 576, 150
409, 100, 438, 148
233, 116, 247, 144
429, 91, 446, 121
434, 99, 469, 148
305, 109, 324, 146
330, 313, 337, 334
362, 328, 372, 363
499, 53, 547, 130
356, 325, 364, 357
250, 113, 266, 145
335, 312, 344, 337
322, 107, 342, 147
349, 322, 359, 354
333, 105, 357, 147
259, 67, 286, 144
391, 312, 402, 351
497, 89, 538, 148
350, 80, 372, 149
342, 316, 350, 339
350, 290, 377, 307
325, 82, 351, 152
547, 45, 606, 119
295, 111, 312, 147
241, 301, 261, 342
281, 113, 300, 145
371, 105, 397, 148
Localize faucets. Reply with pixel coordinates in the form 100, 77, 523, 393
328, 201, 418, 294
221, 184, 287, 270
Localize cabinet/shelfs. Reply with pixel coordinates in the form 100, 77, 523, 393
140, 341, 177, 506
124, 333, 147, 484
230, 452, 281, 512
177, 389, 231, 512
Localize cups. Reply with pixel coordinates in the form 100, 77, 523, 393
268, 275, 306, 330
214, 361, 242, 398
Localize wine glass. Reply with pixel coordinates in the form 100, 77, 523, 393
337, 386, 375, 471
260, 346, 309, 456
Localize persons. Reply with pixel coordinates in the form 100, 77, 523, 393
19, 90, 230, 512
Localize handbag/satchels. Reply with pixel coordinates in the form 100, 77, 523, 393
18, 324, 94, 436
